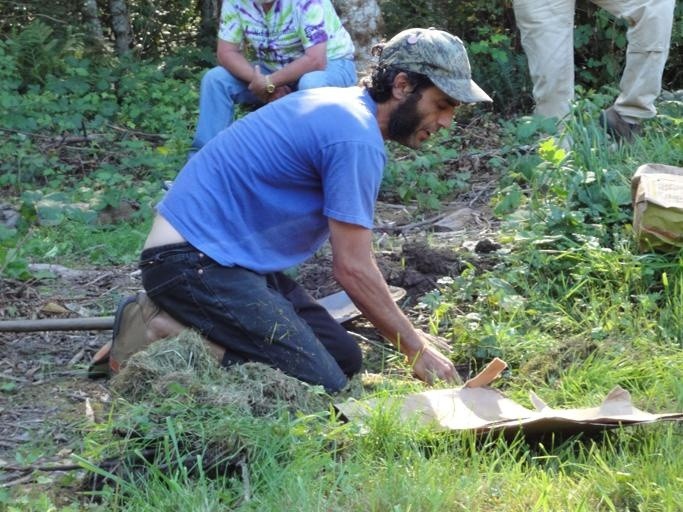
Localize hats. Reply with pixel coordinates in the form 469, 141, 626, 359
379, 27, 494, 103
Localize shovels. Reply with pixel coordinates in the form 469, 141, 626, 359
0, 284, 407, 332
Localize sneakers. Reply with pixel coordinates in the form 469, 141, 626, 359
88, 290, 160, 380
600, 107, 640, 145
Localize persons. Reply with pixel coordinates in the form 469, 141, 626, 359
512, 0, 675, 154
